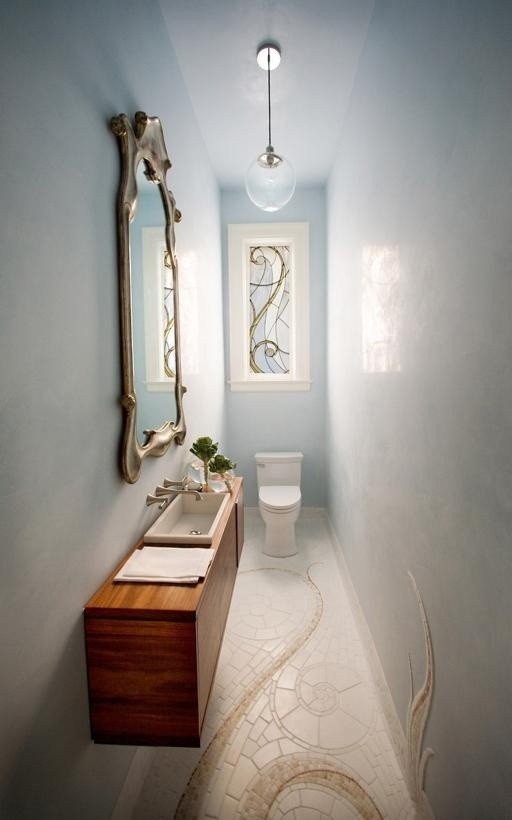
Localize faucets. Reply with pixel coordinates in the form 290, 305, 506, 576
156, 486, 203, 501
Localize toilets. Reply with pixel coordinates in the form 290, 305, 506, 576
254, 451, 303, 558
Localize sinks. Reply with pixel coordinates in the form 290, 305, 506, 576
143, 491, 230, 544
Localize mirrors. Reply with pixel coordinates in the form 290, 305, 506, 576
109, 110, 188, 485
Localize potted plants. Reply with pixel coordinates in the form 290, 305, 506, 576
189, 435, 235, 494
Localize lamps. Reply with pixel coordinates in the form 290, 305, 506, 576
244, 43, 296, 214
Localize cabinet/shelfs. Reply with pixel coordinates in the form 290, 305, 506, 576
235, 485, 244, 568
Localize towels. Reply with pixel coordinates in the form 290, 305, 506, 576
112, 545, 217, 585
